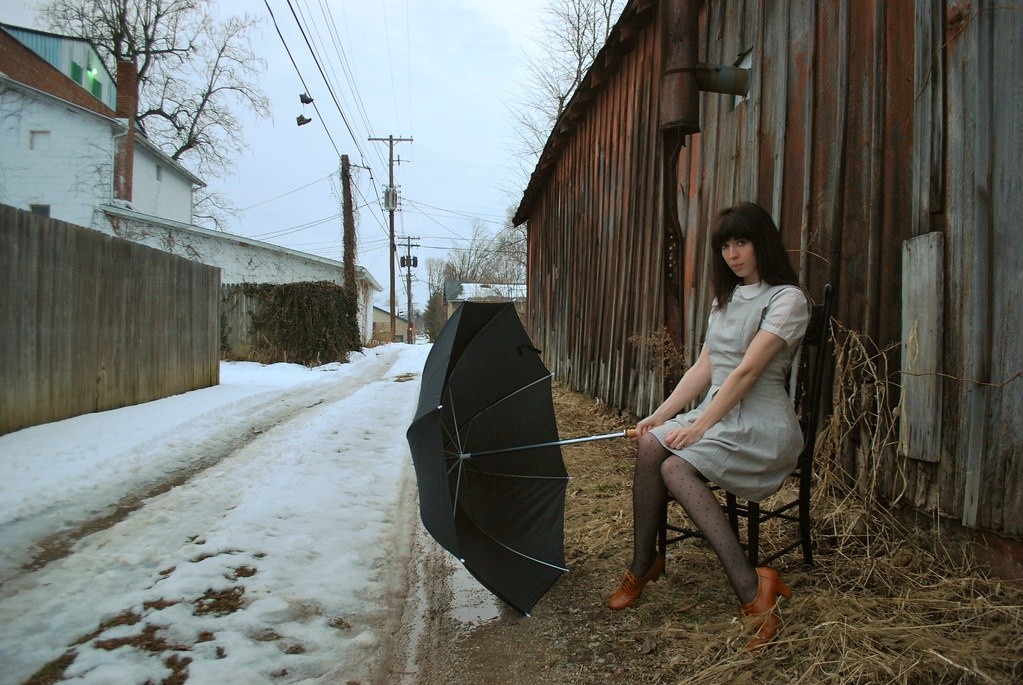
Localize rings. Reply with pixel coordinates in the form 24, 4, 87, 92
680, 442, 684, 450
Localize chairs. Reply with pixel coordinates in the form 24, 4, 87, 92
655, 284, 833, 579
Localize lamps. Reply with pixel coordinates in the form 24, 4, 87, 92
87, 66, 97, 74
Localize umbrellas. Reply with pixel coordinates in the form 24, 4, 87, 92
404, 300, 658, 616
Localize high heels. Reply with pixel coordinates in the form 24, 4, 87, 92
608, 551, 664, 609
742, 567, 790, 653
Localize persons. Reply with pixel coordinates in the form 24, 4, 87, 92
605, 204, 814, 651
